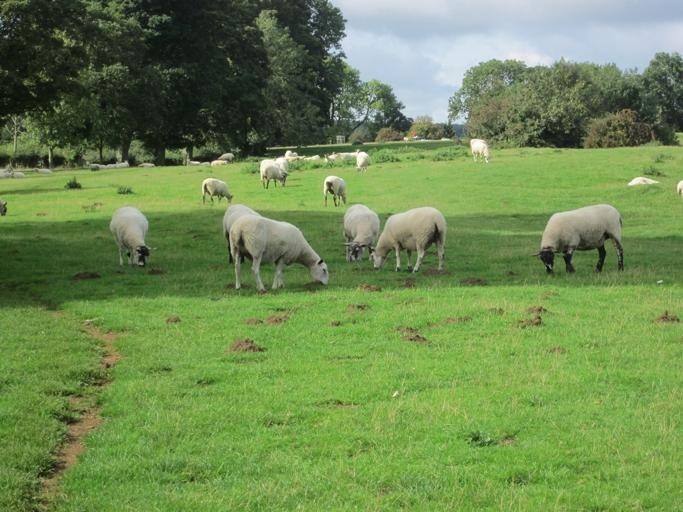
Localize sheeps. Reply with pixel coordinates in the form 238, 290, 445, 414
340, 204, 381, 263
260, 159, 290, 190
471, 136, 488, 163
324, 176, 347, 207
368, 207, 447, 273
202, 178, 233, 205
228, 214, 329, 293
189, 153, 235, 167
275, 148, 370, 173
530, 203, 624, 274
109, 206, 152, 267
222, 204, 263, 264
